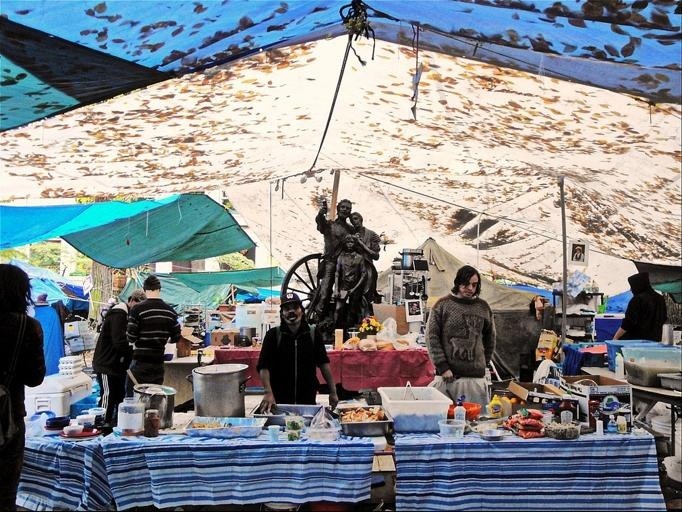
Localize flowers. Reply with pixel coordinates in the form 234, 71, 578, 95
357, 316, 384, 337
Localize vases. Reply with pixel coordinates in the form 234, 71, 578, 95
366, 334, 376, 342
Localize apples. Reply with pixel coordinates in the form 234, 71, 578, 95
520, 401, 526, 408
511, 397, 517, 404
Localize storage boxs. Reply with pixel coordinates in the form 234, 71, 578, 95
377, 386, 454, 432
559, 374, 633, 432
604, 339, 659, 374
595, 313, 625, 342
621, 347, 682, 386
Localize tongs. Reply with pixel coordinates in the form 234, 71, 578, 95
402, 380, 419, 401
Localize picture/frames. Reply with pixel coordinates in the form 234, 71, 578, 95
567, 240, 589, 266
405, 299, 424, 322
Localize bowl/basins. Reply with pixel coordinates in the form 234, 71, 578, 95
449, 401, 482, 422
438, 419, 466, 440
45, 407, 106, 436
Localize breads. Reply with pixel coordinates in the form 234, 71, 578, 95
343, 406, 384, 422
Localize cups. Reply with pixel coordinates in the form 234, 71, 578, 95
268, 424, 280, 444
251, 336, 258, 348
334, 326, 343, 350
617, 415, 628, 434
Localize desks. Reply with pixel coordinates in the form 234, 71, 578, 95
162, 343, 214, 406
215, 348, 341, 388
18, 418, 112, 510
341, 350, 429, 397
580, 366, 682, 455
102, 424, 388, 512
385, 418, 666, 512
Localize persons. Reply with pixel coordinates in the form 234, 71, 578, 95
125, 274, 181, 398
349, 212, 381, 307
332, 233, 367, 337
256, 292, 340, 413
612, 272, 667, 423
311, 198, 352, 323
572, 245, 586, 262
90, 289, 148, 427
0, 263, 48, 512
421, 264, 496, 415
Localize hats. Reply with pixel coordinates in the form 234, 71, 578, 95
131, 289, 146, 300
280, 293, 299, 307
143, 276, 161, 291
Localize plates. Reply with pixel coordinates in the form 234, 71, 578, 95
479, 428, 504, 441
651, 415, 681, 435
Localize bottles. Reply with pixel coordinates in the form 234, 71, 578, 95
453, 401, 467, 421
488, 394, 526, 418
615, 352, 625, 380
346, 326, 361, 339
561, 406, 574, 425
115, 396, 160, 439
596, 419, 604, 436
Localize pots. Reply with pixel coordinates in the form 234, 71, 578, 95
238, 326, 256, 338
134, 382, 177, 430
187, 363, 253, 417
